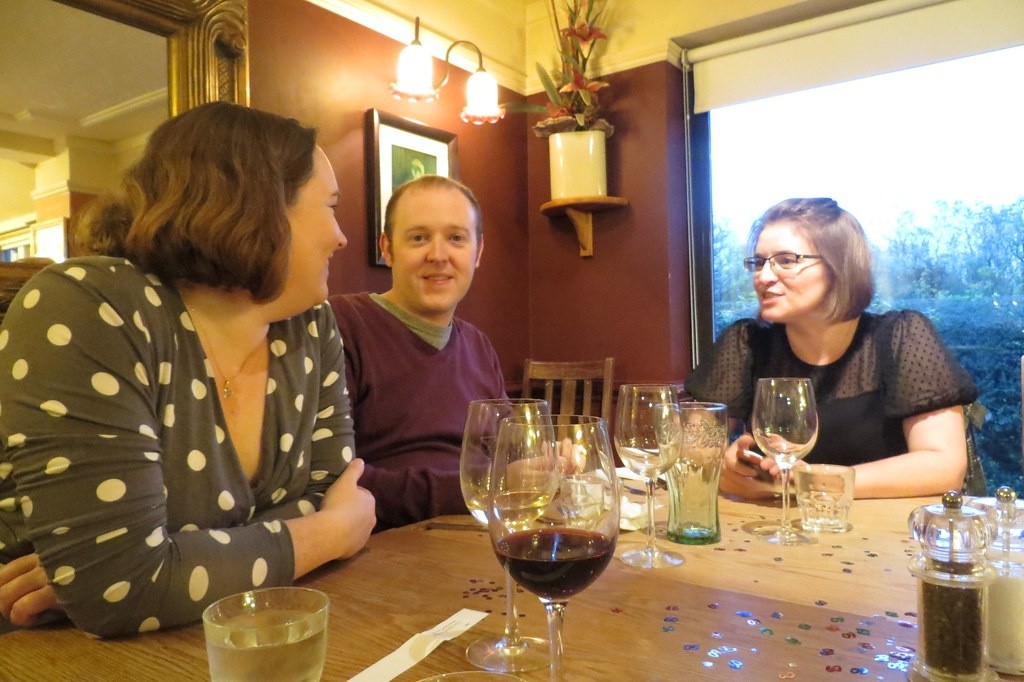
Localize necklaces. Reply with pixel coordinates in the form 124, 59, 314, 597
180, 291, 265, 400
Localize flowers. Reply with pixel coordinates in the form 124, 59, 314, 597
499, 0, 615, 140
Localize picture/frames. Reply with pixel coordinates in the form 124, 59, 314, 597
366, 108, 458, 268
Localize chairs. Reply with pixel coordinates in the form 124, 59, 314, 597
522, 357, 616, 472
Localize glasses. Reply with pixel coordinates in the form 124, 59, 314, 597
744, 252, 824, 269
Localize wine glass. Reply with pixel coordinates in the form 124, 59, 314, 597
614, 384, 685, 569
753, 377, 819, 546
460, 398, 555, 672
489, 413, 620, 682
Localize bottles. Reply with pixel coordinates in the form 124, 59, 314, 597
906, 490, 1000, 682
968, 486, 1024, 678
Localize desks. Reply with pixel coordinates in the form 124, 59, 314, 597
0, 471, 1024, 682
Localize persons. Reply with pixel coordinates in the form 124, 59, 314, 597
679, 198, 979, 502
327, 174, 588, 534
0, 100, 377, 639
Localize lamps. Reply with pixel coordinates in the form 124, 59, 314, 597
386, 16, 506, 126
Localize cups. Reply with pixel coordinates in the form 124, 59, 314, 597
648, 404, 727, 544
202, 587, 330, 682
792, 465, 856, 533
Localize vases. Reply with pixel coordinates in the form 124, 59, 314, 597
549, 130, 606, 201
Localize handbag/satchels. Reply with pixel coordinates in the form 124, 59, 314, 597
964, 400, 986, 498
